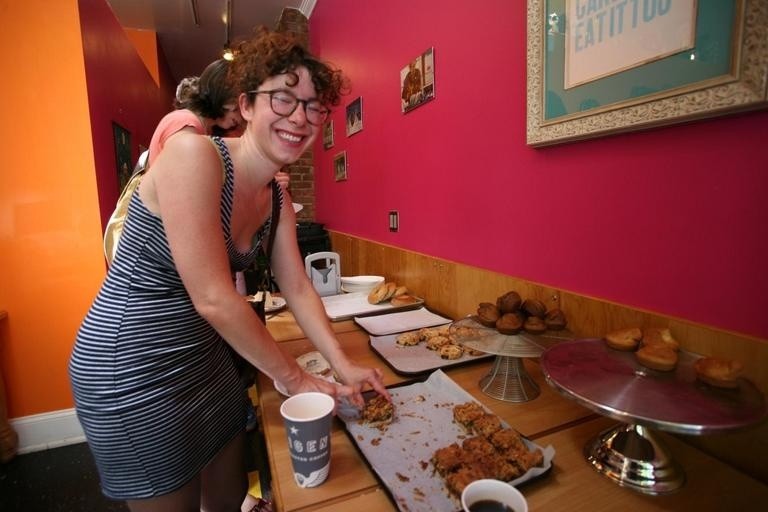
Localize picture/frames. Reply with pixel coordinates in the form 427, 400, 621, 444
332, 151, 347, 183
525, 0, 768, 151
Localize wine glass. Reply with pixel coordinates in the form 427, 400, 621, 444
455, 322, 582, 398
545, 338, 765, 496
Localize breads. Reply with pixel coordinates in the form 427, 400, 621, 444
431, 400, 543, 497
694, 357, 744, 389
604, 325, 679, 371
396, 291, 567, 361
361, 394, 393, 421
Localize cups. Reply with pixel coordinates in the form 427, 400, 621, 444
279, 392, 334, 489
459, 478, 528, 512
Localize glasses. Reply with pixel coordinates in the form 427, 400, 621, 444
248, 89, 331, 126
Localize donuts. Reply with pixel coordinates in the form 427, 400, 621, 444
367, 283, 415, 307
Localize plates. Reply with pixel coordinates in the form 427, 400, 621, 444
263, 297, 285, 313
274, 351, 341, 397
340, 274, 386, 294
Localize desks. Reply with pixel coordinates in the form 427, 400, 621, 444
250, 283, 767, 512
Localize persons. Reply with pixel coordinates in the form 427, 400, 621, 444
402, 59, 421, 105
66, 23, 390, 511
